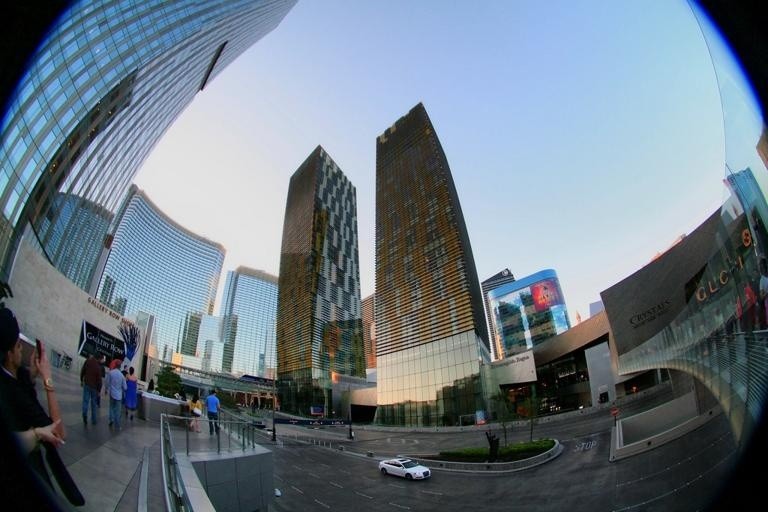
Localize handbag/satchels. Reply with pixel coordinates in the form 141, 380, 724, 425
194, 406, 201, 416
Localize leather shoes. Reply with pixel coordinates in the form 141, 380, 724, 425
189, 426, 214, 435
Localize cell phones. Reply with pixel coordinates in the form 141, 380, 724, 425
35, 338, 42, 360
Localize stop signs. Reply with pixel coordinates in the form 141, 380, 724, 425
611, 408, 618, 418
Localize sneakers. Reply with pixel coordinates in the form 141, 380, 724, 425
82, 411, 133, 431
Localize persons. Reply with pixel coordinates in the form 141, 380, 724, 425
714, 257, 768, 332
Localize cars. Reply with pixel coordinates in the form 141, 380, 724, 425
538, 403, 563, 413
377, 458, 433, 480
